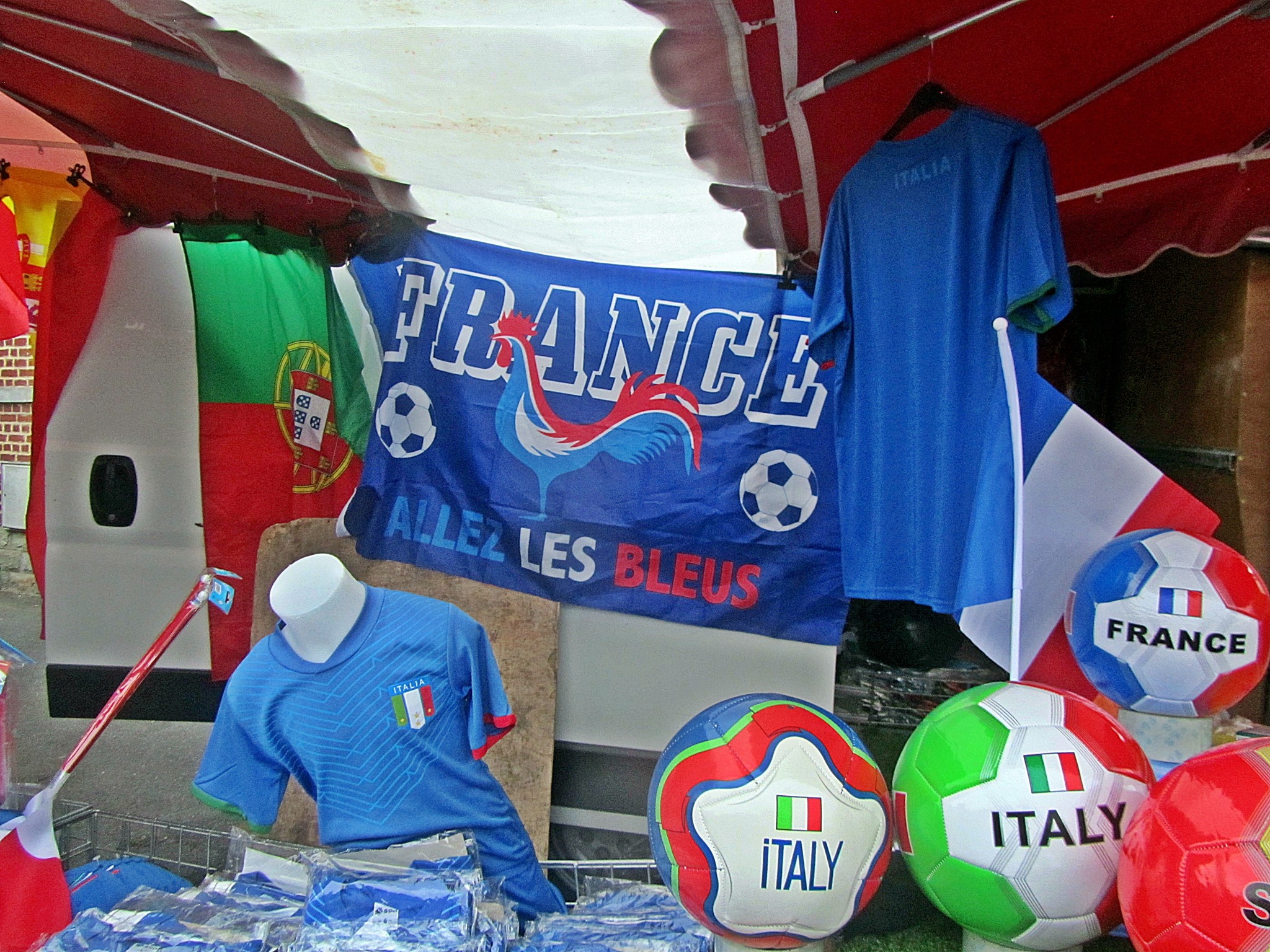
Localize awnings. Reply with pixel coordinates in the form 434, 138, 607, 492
0, 1, 435, 270
628, 0, 1270, 280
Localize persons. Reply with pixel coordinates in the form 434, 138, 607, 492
224, 553, 567, 935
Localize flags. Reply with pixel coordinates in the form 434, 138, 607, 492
335, 220, 853, 646
949, 353, 1220, 701
172, 219, 375, 685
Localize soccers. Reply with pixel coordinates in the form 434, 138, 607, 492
643, 690, 892, 945
1063, 526, 1270, 718
1115, 736, 1267, 952
888, 679, 1160, 951
